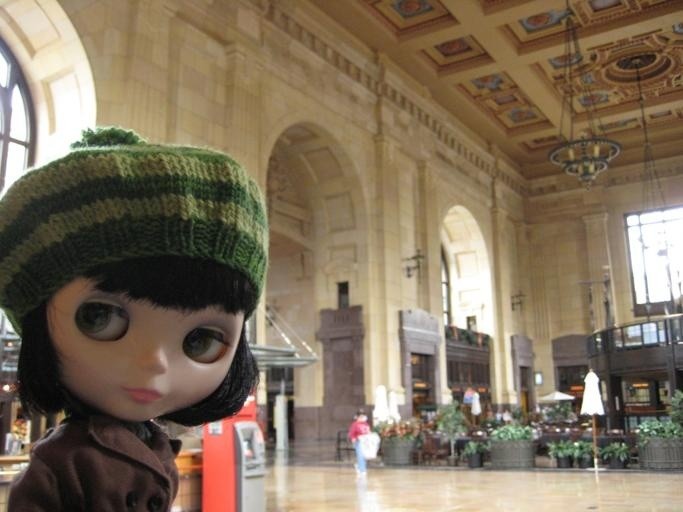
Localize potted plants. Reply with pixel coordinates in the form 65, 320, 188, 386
375, 389, 683, 471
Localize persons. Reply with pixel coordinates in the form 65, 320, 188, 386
348, 408, 372, 477
1, 125, 271, 512
482, 403, 598, 457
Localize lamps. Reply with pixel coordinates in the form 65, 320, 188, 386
634, 62, 678, 259
549, 1, 622, 190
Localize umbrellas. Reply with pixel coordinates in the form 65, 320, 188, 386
469, 391, 483, 425
535, 390, 575, 406
578, 367, 607, 467
